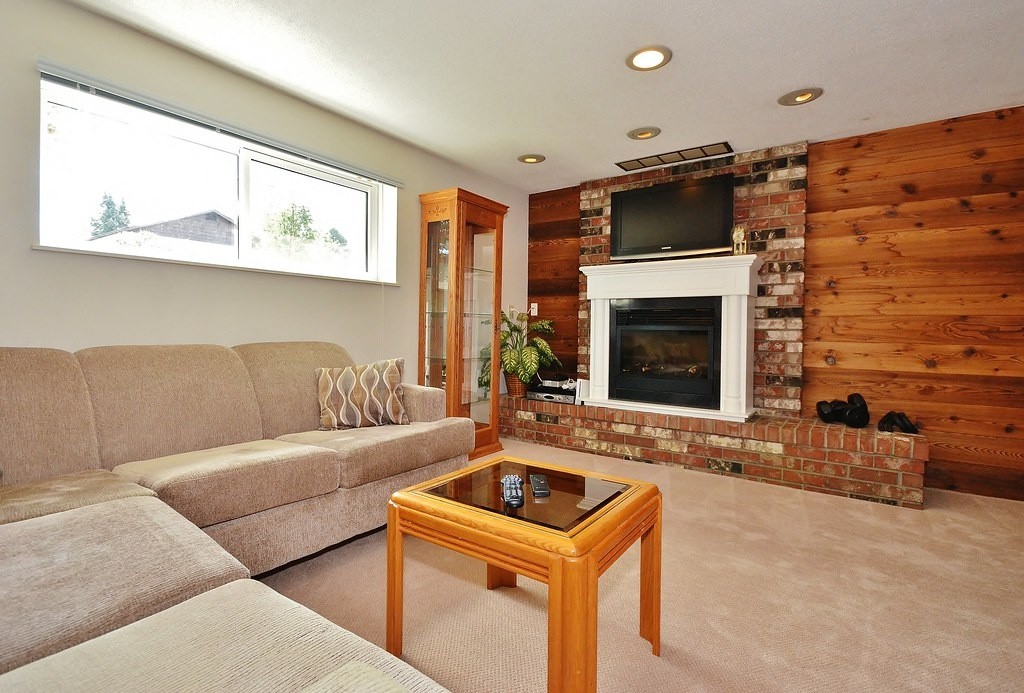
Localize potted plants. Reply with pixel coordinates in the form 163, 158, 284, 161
478, 311, 563, 399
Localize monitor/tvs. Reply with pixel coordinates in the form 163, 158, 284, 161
610, 172, 736, 263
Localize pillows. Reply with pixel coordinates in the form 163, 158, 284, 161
314, 358, 409, 432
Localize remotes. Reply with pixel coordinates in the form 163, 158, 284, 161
530, 474, 550, 498
502, 474, 525, 507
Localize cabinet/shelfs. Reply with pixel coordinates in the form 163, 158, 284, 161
419, 188, 510, 461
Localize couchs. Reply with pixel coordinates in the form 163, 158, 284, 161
1, 342, 475, 693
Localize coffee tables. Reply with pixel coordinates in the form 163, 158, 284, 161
387, 456, 662, 693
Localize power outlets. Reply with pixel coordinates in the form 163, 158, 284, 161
531, 303, 538, 316
509, 305, 514, 319
